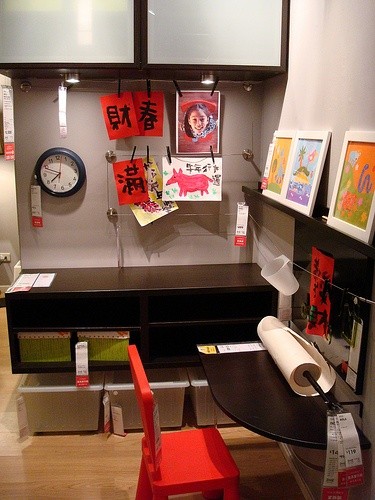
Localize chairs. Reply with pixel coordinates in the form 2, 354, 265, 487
127, 344, 242, 500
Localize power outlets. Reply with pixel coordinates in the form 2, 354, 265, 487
0, 253, 11, 262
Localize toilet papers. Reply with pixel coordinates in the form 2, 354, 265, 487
256, 315, 337, 396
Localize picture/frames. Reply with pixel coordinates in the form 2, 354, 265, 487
325, 128, 375, 247
261, 129, 298, 203
280, 130, 332, 217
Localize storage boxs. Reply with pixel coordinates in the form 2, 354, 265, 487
188, 367, 241, 426
16, 331, 73, 363
17, 370, 105, 434
77, 330, 132, 363
106, 367, 191, 433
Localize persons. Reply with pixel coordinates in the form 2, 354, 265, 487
185, 104, 211, 138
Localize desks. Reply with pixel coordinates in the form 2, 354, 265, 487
4, 261, 280, 376
196, 341, 372, 451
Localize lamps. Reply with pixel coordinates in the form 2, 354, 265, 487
65, 73, 80, 84
201, 74, 215, 87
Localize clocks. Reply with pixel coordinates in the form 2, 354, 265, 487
35, 147, 87, 198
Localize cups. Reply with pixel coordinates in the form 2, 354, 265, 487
261, 255, 300, 296
